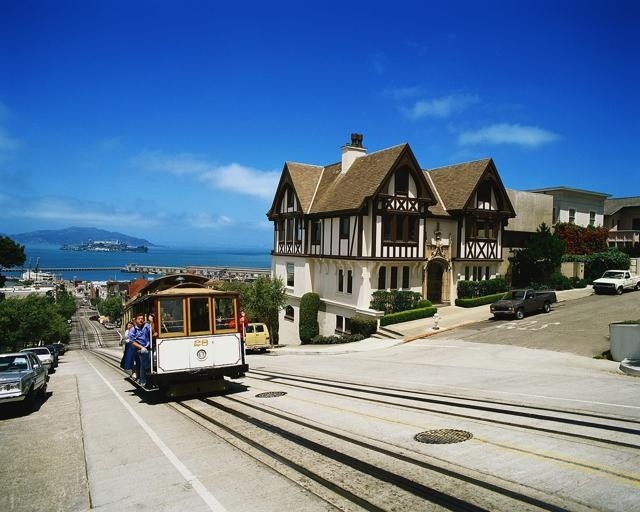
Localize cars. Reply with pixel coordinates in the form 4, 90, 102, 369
0, 339, 67, 410
79, 303, 122, 329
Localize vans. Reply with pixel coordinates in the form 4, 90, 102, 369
244, 322, 272, 354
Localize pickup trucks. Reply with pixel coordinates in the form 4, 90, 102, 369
489, 287, 557, 321
592, 269, 640, 295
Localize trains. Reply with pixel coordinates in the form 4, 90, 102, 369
118, 272, 249, 401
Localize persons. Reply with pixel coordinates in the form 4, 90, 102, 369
228, 309, 248, 364
120, 312, 158, 386
216, 313, 227, 330
